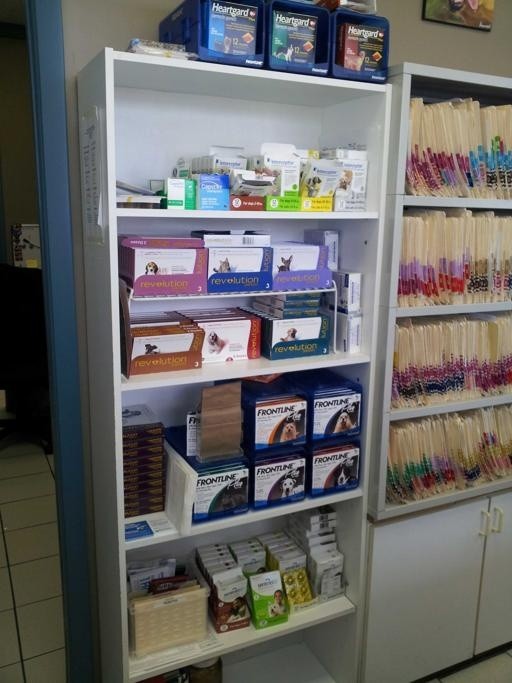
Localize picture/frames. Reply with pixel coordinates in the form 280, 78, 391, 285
421, 0, 494, 31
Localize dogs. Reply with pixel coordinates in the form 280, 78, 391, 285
143, 343, 160, 355
219, 493, 245, 511
212, 257, 233, 273
344, 49, 366, 70
207, 331, 220, 354
334, 466, 355, 490
333, 413, 353, 434
283, 328, 298, 342
277, 254, 294, 272
144, 261, 160, 276
272, 44, 296, 63
279, 477, 297, 499
339, 177, 349, 191
279, 420, 298, 442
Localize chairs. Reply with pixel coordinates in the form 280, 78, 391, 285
1, 262, 53, 455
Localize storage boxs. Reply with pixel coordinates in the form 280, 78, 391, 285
158, 0, 388, 84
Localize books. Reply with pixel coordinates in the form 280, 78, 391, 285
398, 208, 512, 308
402, 98, 512, 200
389, 310, 512, 410
384, 405, 512, 505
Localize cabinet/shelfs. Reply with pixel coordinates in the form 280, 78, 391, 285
362, 58, 512, 683
75, 46, 393, 683
